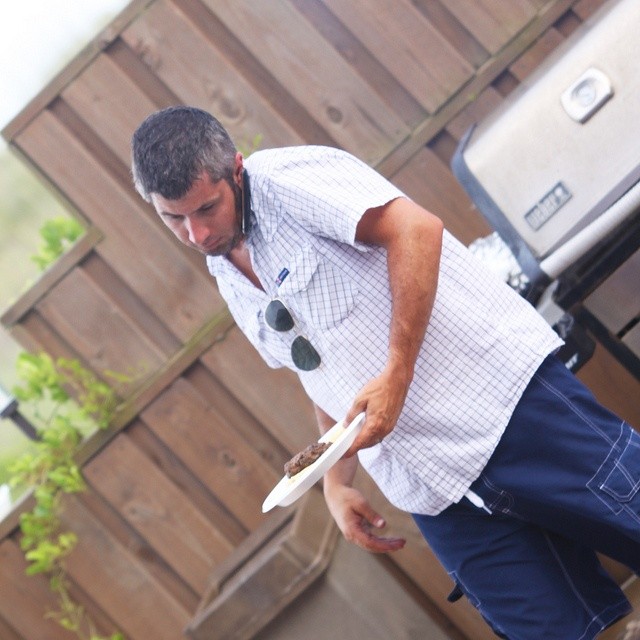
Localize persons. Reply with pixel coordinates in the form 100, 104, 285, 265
133, 107, 638, 640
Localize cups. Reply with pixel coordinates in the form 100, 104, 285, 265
263, 299, 321, 371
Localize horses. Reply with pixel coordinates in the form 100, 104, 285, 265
240, 167, 250, 237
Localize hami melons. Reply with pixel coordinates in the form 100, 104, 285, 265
285, 442, 332, 478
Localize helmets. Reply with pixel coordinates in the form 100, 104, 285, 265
262, 409, 367, 516
